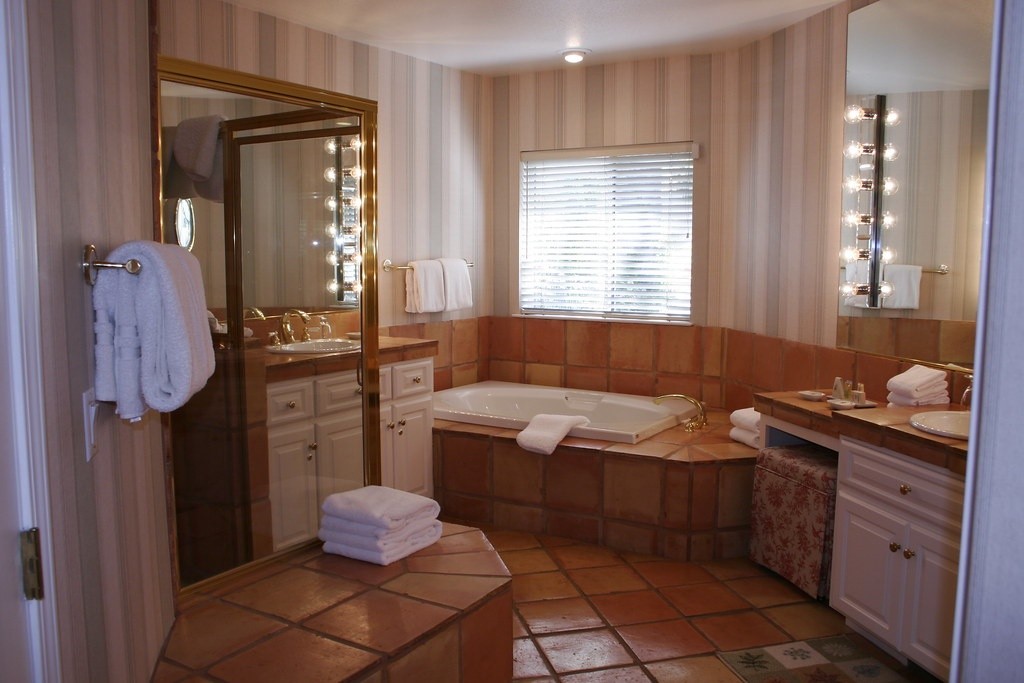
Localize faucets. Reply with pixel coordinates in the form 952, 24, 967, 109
652, 393, 706, 430
280, 309, 312, 343
243, 306, 266, 321
960, 374, 974, 406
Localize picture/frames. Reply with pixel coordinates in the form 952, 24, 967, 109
175, 197, 195, 252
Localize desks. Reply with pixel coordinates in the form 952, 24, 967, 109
751, 388, 888, 452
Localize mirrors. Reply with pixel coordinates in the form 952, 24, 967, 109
838, 0, 996, 320
162, 95, 362, 323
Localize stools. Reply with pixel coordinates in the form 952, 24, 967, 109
749, 442, 838, 606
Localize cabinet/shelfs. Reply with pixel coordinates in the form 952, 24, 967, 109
266, 355, 435, 553
828, 433, 967, 682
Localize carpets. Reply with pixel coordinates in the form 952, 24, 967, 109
716, 632, 910, 683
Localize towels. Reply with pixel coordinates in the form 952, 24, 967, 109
728, 406, 761, 449
844, 260, 882, 310
886, 363, 951, 408
173, 113, 229, 204
516, 413, 592, 455
438, 258, 474, 312
91, 239, 217, 424
317, 484, 443, 567
882, 264, 923, 310
404, 259, 445, 314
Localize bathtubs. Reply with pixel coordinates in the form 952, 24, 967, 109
432, 380, 706, 445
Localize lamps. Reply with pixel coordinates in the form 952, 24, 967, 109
559, 48, 592, 63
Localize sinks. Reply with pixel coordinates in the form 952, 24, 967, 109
909, 410, 970, 441
265, 338, 361, 353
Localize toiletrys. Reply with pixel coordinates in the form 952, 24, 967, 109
832, 377, 865, 404
